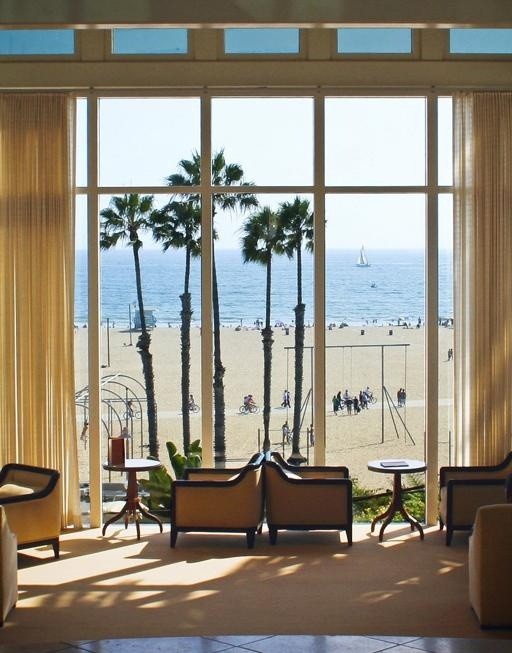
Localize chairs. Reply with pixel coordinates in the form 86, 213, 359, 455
439, 451, 512, 547
170, 452, 266, 549
0, 505, 19, 628
468, 502, 512, 631
263, 450, 352, 546
0, 463, 62, 559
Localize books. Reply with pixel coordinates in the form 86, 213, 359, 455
381, 460, 410, 467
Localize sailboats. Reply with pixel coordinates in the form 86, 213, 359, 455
356, 244, 370, 266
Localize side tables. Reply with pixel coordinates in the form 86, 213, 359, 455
366, 458, 427, 542
102, 458, 163, 541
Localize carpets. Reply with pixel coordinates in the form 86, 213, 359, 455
1, 524, 512, 652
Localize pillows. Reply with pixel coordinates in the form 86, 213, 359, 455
0, 484, 35, 499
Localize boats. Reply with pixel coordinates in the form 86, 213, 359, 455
371, 280, 377, 288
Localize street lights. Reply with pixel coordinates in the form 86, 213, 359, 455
128, 299, 137, 345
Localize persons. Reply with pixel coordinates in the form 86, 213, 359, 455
282, 390, 292, 408
188, 394, 195, 412
447, 348, 454, 361
309, 423, 315, 447
243, 394, 254, 414
125, 399, 135, 415
332, 386, 374, 416
397, 388, 407, 408
281, 421, 291, 443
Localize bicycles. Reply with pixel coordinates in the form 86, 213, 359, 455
123, 408, 142, 419
181, 401, 200, 413
239, 400, 261, 414
367, 391, 378, 404
